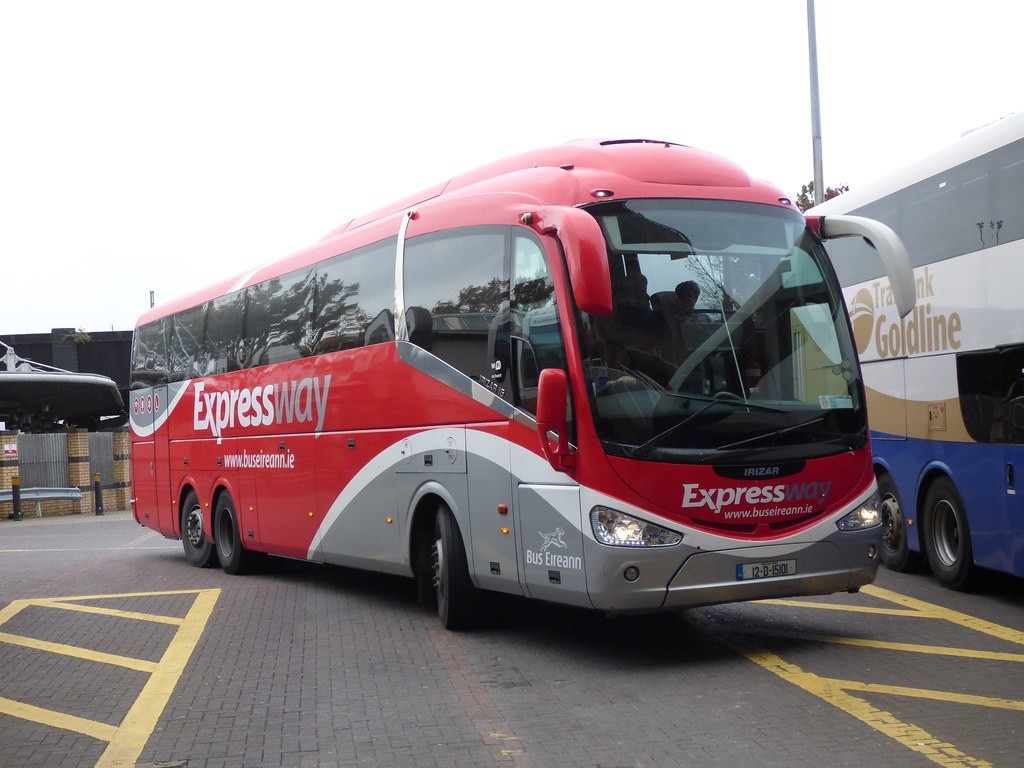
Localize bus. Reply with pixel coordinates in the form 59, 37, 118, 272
720, 110, 1024, 594
129, 140, 916, 632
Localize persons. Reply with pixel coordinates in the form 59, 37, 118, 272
627, 271, 652, 310
664, 280, 750, 401
